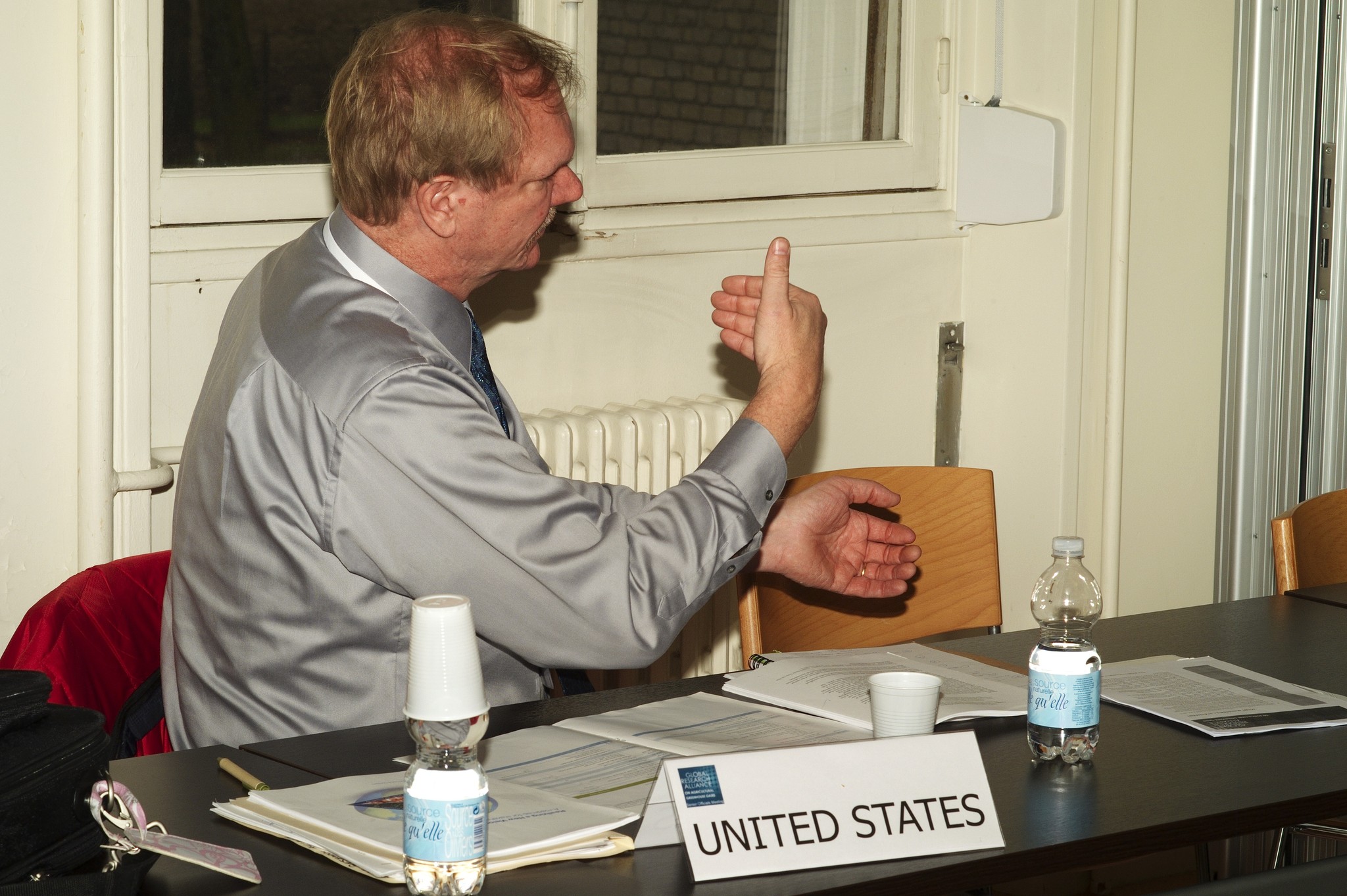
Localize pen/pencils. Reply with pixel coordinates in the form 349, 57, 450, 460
216, 756, 269, 792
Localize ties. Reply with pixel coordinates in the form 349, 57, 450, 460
465, 308, 511, 441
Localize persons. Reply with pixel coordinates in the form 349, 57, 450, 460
159, 8, 923, 750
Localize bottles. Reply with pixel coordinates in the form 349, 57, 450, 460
1027, 536, 1103, 763
402, 711, 489, 896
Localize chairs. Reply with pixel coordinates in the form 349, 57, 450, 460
1264, 489, 1346, 870
0, 549, 176, 760
739, 466, 1004, 670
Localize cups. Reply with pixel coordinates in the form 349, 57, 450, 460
402, 594, 491, 722
867, 672, 944, 738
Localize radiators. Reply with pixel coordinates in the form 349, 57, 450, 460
519, 397, 751, 696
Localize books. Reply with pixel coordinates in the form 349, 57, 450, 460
211, 770, 640, 884
721, 641, 1029, 730
394, 690, 873, 814
1098, 655, 1347, 737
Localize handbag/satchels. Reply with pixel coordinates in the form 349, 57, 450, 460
0, 670, 262, 896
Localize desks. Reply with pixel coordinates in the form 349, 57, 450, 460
109, 581, 1346, 895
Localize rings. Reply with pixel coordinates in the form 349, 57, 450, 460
861, 563, 867, 577
